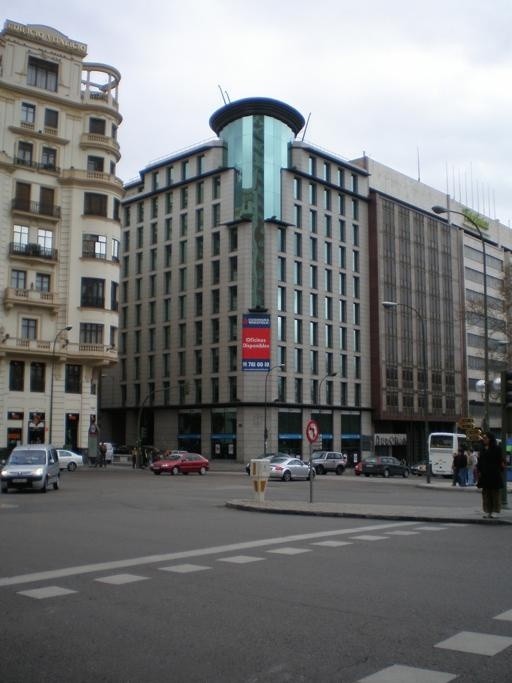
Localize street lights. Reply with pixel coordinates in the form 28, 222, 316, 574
432, 205, 489, 483
49, 326, 73, 443
382, 301, 432, 483
265, 364, 337, 453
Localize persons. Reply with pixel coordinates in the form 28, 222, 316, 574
100, 440, 108, 467
452, 431, 505, 518
130, 445, 162, 469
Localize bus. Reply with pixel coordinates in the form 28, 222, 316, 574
428, 432, 466, 478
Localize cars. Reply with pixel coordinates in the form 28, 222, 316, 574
0, 444, 84, 493
246, 451, 428, 481
149, 452, 210, 475
99, 442, 113, 463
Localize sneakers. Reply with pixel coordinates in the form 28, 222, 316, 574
482, 511, 498, 519
